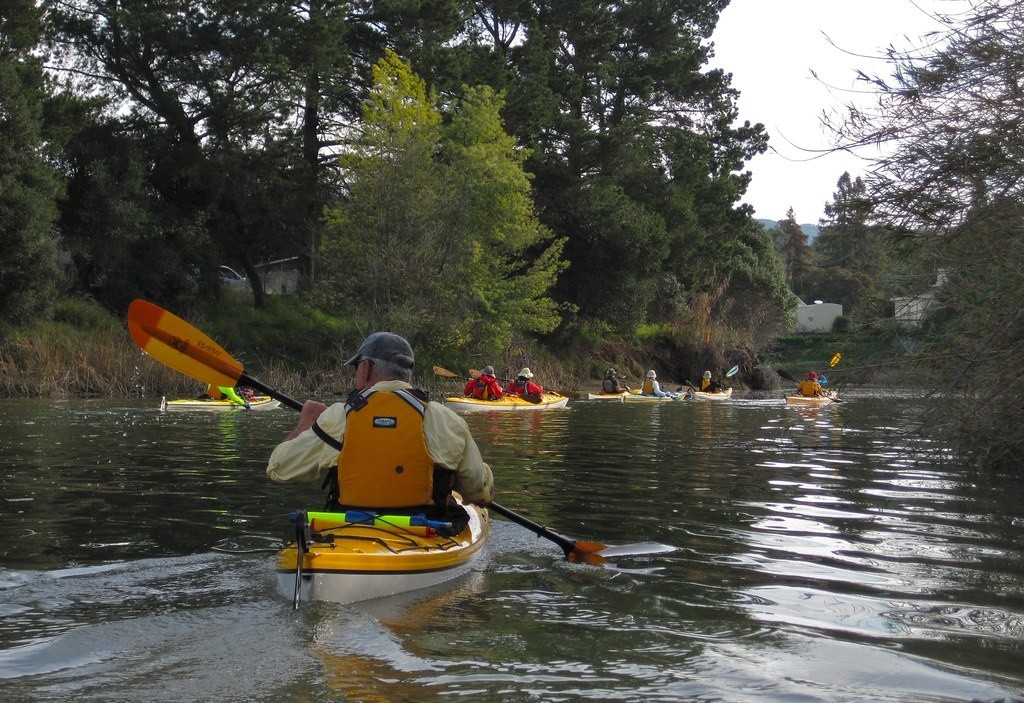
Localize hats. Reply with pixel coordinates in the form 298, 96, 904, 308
805, 371, 819, 380
703, 371, 711, 378
481, 366, 495, 377
343, 331, 415, 370
607, 369, 617, 374
646, 369, 657, 377
518, 368, 533, 378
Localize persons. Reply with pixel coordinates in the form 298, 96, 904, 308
207, 384, 251, 411
601, 368, 824, 398
466, 367, 545, 404
266, 332, 493, 541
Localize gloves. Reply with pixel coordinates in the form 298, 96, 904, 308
245, 403, 250, 410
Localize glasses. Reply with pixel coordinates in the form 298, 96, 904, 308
354, 358, 375, 370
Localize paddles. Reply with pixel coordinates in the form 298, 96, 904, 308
127, 299, 608, 561
433, 366, 542, 404
819, 353, 842, 374
777, 369, 843, 402
715, 365, 739, 383
237, 387, 248, 402
469, 369, 581, 399
627, 390, 639, 396
666, 395, 681, 400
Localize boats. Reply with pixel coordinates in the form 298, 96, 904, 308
275, 489, 490, 613
588, 390, 643, 400
785, 388, 839, 406
159, 395, 282, 413
620, 391, 686, 403
440, 391, 569, 412
692, 386, 734, 401
